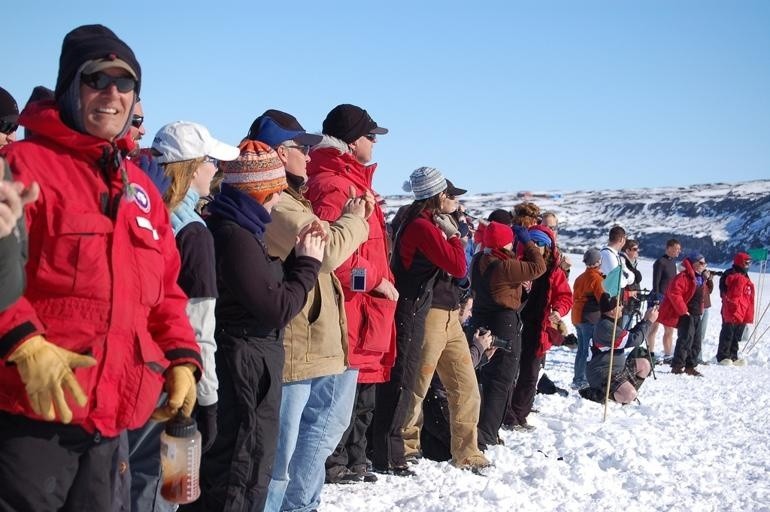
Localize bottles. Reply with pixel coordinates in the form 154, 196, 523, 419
161, 411, 203, 505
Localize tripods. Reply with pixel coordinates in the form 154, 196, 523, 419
628, 311, 656, 381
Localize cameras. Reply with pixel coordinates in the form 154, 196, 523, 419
351, 268, 368, 292
476, 328, 513, 353
628, 291, 647, 307
709, 270, 722, 277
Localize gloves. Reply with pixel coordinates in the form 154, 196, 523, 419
8, 335, 96, 424
434, 213, 461, 239
150, 362, 197, 422
513, 224, 531, 244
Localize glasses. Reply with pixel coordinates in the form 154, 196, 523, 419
131, 116, 144, 127
82, 73, 137, 92
747, 262, 752, 266
289, 144, 311, 155
446, 195, 455, 200
632, 248, 639, 251
0, 121, 18, 133
363, 133, 376, 139
533, 241, 545, 246
698, 260, 704, 265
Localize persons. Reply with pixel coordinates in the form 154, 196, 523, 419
715, 251, 755, 367
0, 22, 661, 511
695, 269, 714, 366
645, 239, 683, 365
656, 250, 707, 379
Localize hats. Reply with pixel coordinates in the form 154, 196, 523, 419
249, 110, 323, 146
0, 87, 20, 123
80, 57, 139, 82
529, 224, 555, 240
323, 104, 388, 143
599, 293, 617, 312
537, 373, 568, 396
446, 178, 467, 195
483, 222, 514, 249
151, 121, 240, 163
583, 247, 602, 268
223, 139, 288, 204
689, 249, 705, 263
409, 167, 448, 200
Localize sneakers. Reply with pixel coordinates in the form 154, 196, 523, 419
334, 455, 423, 483
462, 452, 495, 474
652, 356, 747, 365
571, 384, 585, 389
502, 419, 536, 435
671, 367, 700, 376
